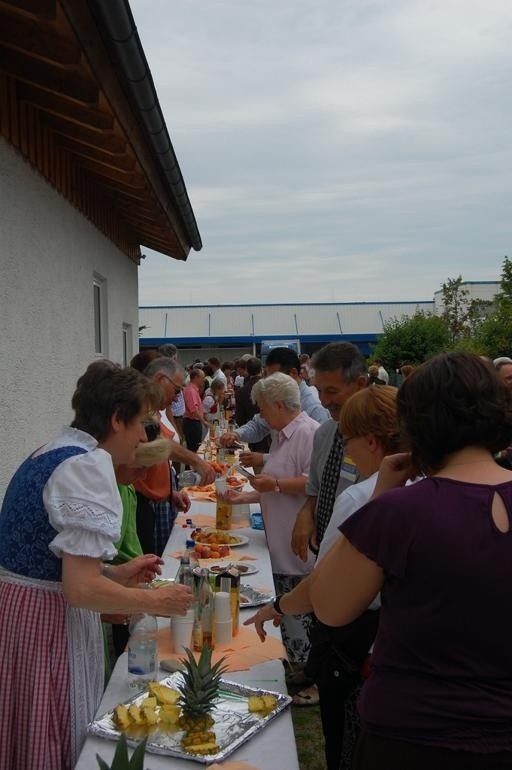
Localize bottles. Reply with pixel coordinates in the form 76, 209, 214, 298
127, 582, 160, 691
175, 405, 251, 530
168, 518, 259, 653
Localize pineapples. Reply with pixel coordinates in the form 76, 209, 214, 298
112, 643, 279, 755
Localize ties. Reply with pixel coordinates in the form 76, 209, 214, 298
316, 424, 342, 546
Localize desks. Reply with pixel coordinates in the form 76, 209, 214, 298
74, 425, 300, 770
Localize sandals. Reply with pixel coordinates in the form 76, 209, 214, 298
293, 684, 321, 706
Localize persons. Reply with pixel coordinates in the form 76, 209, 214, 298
0, 341, 511, 770
